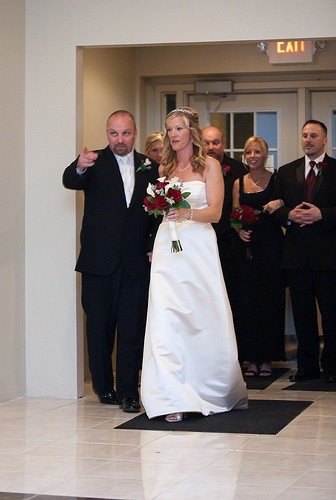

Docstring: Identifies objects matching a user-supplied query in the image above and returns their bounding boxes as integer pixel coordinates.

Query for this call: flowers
[143,176,191,253]
[231,206,262,259]
[316,162,328,174]
[222,165,231,176]
[136,157,152,172]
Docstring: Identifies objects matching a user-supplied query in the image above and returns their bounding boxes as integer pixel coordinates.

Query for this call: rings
[174,214,176,218]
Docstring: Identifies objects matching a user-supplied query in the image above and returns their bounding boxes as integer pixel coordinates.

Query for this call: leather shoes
[119,399,140,412]
[97,389,117,404]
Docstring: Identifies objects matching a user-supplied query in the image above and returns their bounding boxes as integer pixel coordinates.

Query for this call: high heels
[165,412,188,422]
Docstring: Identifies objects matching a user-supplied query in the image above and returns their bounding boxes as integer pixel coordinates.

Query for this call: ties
[120,156,131,204]
[306,161,318,202]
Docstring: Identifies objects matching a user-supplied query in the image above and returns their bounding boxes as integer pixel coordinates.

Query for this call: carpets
[114,400,314,434]
[241,367,291,390]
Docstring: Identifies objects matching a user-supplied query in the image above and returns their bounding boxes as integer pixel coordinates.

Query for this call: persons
[232,136,285,377]
[138,130,167,371]
[140,106,249,422]
[61,109,164,414]
[276,119,336,383]
[199,124,250,313]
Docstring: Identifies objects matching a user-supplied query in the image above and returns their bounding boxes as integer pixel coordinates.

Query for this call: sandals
[244,361,273,377]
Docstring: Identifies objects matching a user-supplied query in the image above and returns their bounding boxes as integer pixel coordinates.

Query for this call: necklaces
[174,162,191,172]
[249,173,265,185]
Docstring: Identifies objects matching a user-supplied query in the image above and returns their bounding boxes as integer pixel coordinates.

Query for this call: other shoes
[289,370,320,382]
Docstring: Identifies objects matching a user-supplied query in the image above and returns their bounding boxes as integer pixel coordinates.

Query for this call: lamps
[257,40,327,65]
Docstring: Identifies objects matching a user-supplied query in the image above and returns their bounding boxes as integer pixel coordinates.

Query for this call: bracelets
[187,208,194,220]
